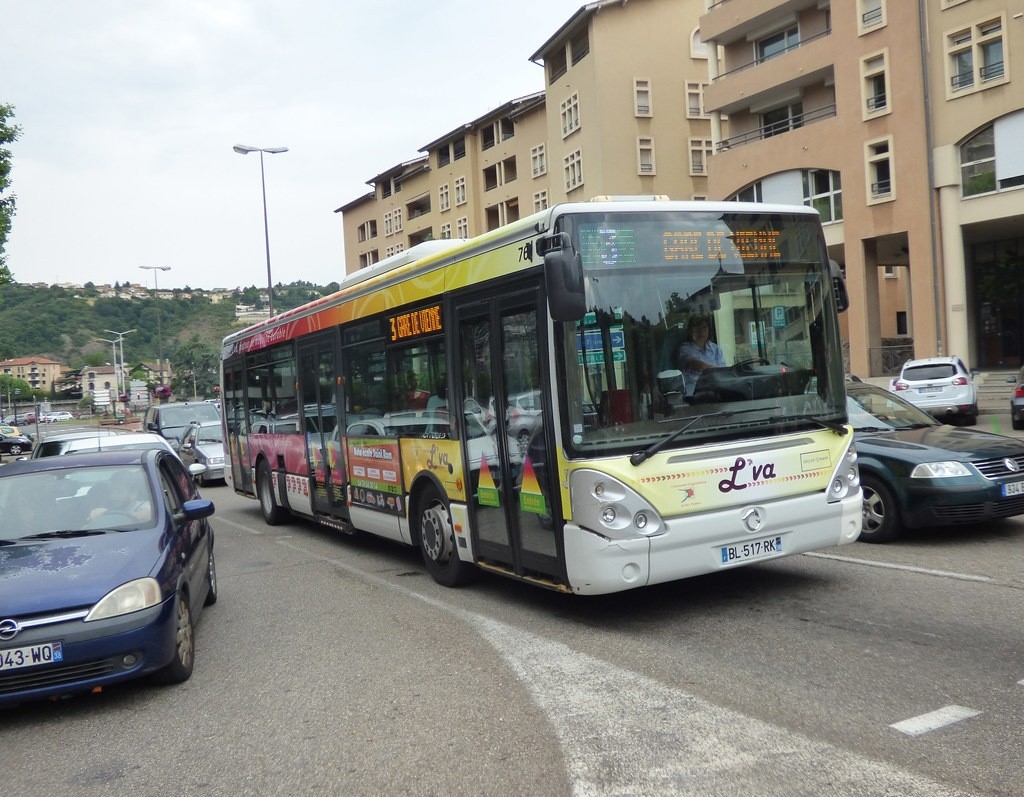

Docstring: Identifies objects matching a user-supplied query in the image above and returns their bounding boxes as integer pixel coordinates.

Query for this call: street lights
[233,144,289,401]
[104,329,136,394]
[139,265,171,386]
[95,338,124,402]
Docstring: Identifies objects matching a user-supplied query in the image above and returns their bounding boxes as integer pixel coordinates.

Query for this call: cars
[1,411,74,425]
[228,389,545,496]
[202,400,222,419]
[803,373,1024,544]
[0,423,33,455]
[0,450,217,706]
[16,429,206,482]
[1006,366,1024,430]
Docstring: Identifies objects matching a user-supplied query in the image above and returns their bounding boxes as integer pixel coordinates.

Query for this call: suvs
[178,421,224,486]
[886,354,980,426]
[143,402,222,455]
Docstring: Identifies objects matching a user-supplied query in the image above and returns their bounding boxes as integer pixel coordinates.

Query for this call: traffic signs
[577,331,625,352]
[577,350,627,364]
[574,309,623,329]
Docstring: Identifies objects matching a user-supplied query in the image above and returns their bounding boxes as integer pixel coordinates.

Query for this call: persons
[89,472,152,523]
[426,373,450,435]
[406,371,427,409]
[675,313,727,405]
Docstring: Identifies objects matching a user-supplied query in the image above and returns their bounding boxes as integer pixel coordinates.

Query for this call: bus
[219,195,862,598]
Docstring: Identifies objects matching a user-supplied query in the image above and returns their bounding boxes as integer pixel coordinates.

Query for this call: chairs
[347,394,485,442]
